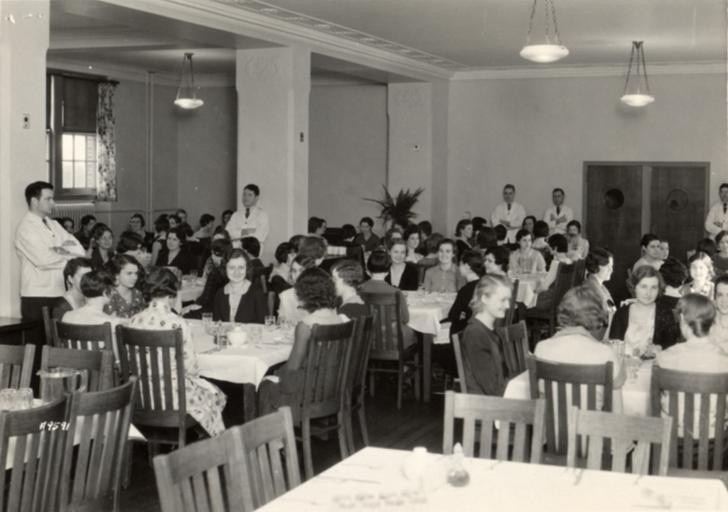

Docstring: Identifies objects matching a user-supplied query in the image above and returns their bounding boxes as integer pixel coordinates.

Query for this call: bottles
[402,446,438,478]
[448,443,470,488]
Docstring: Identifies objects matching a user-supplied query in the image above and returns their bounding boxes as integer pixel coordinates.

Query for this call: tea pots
[36,366,88,430]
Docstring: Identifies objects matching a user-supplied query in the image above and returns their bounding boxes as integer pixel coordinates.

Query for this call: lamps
[174,51,205,110]
[518,0,568,65]
[618,41,655,110]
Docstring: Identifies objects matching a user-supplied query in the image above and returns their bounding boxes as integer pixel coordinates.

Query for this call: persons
[47,184,363,471]
[15,184,87,398]
[345,184,727,472]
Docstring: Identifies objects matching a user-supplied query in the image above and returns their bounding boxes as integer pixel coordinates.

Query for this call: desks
[494,354,727,462]
[254,446,727,511]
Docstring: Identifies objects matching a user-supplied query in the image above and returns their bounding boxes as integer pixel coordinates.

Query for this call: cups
[0,386,35,411]
[609,339,640,381]
[201,310,294,349]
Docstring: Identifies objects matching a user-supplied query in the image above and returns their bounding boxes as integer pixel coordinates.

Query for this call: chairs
[153,426,254,512]
[252,320,349,483]
[308,318,372,447]
[529,354,613,469]
[565,404,676,478]
[116,324,201,494]
[652,364,726,482]
[59,374,137,512]
[0,222,728,392]
[442,390,548,464]
[3,394,64,512]
[236,405,301,510]
[358,290,430,407]
